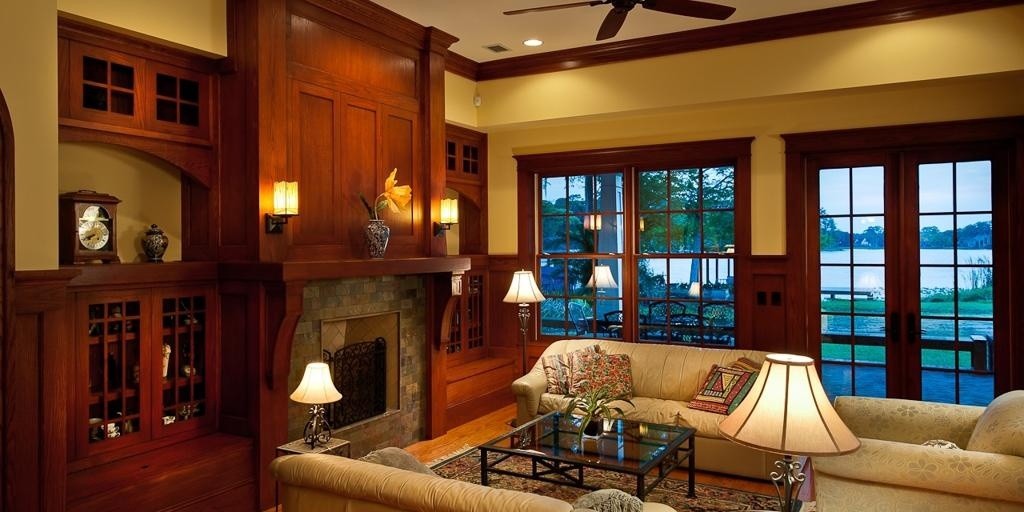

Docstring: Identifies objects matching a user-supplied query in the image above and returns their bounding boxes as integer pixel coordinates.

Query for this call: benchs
[824,329,986,372]
[821,288,873,300]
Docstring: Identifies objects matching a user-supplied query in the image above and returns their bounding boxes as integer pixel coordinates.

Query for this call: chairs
[603,311,647,340]
[697,302,734,345]
[648,299,686,341]
[567,302,606,337]
[664,313,708,343]
[811,387,1023,511]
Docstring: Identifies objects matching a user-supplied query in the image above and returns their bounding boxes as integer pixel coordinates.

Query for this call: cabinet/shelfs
[446,121,488,185]
[445,267,493,370]
[58,8,220,149]
[71,281,218,462]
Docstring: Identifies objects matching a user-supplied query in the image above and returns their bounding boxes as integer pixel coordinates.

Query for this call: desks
[591,317,735,346]
[272,435,352,511]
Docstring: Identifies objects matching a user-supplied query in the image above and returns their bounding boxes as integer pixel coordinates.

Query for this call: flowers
[369,167,413,220]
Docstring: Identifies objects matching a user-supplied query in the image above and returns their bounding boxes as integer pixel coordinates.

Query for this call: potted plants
[562,381,635,447]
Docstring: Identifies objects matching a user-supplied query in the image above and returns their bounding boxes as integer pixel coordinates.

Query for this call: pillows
[566,351,632,400]
[569,487,644,512]
[729,357,762,370]
[686,364,759,416]
[923,438,962,451]
[354,446,442,478]
[540,344,600,395]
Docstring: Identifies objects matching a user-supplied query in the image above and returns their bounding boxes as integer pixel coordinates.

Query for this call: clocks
[58,189,122,264]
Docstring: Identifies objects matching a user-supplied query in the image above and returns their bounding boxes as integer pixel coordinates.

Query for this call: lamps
[639,217,644,232]
[715,353,862,511]
[586,264,618,296]
[588,214,602,231]
[687,281,703,297]
[433,198,458,237]
[263,180,300,232]
[502,268,546,377]
[289,361,345,448]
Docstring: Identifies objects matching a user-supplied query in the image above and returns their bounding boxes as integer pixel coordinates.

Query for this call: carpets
[424,442,817,509]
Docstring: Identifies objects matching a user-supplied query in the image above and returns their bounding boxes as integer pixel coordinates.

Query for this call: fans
[502,0,739,42]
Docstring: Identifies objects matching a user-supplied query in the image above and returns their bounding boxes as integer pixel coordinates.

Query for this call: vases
[362,219,391,260]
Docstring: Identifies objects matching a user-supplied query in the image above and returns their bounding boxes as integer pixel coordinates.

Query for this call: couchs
[268,447,679,512]
[510,337,800,486]
